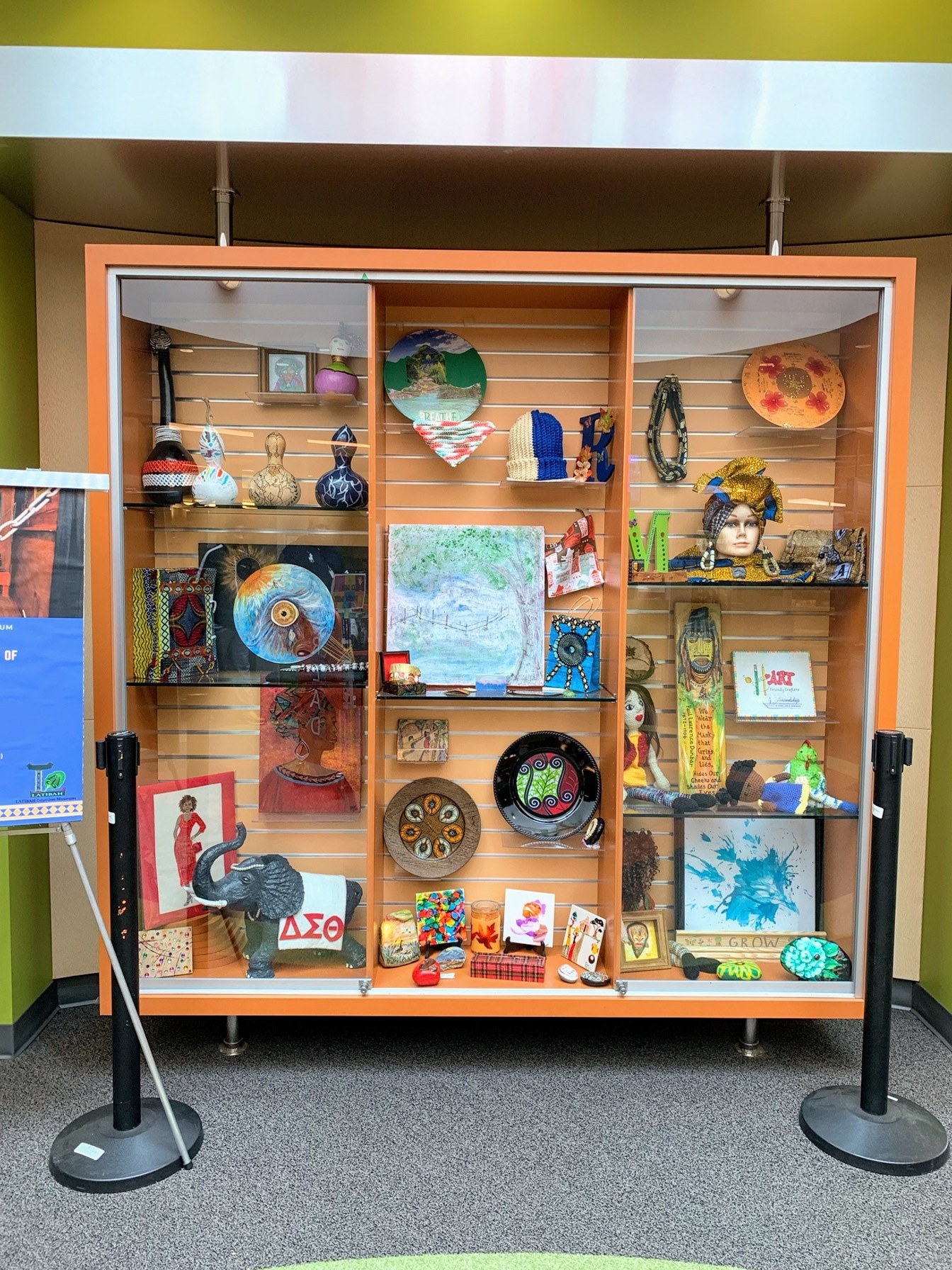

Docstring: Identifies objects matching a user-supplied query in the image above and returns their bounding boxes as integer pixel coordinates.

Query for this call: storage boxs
[471,952,546,983]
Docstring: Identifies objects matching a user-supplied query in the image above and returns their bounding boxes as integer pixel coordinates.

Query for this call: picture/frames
[621,909,672,976]
[258,342,318,393]
[674,806,826,933]
[137,771,240,933]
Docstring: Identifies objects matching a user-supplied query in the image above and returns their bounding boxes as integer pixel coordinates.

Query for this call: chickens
[781,739,827,794]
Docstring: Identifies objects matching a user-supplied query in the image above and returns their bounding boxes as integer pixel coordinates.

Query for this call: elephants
[180,822,367,978]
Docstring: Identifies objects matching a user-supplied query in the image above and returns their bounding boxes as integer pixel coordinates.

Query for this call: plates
[741,342,846,429]
[493,731,602,842]
[384,777,482,878]
[233,564,334,664]
[383,328,486,425]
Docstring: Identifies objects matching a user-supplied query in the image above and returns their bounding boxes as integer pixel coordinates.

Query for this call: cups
[471,900,501,954]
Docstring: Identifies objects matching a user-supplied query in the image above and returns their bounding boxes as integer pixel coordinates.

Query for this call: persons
[716,759,858,815]
[692,456,783,559]
[623,827,722,980]
[623,683,716,811]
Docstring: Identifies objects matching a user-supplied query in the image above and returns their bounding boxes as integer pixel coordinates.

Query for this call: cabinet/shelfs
[84,240,921,1061]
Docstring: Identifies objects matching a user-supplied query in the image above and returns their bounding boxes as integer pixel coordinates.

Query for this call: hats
[506,409,567,480]
